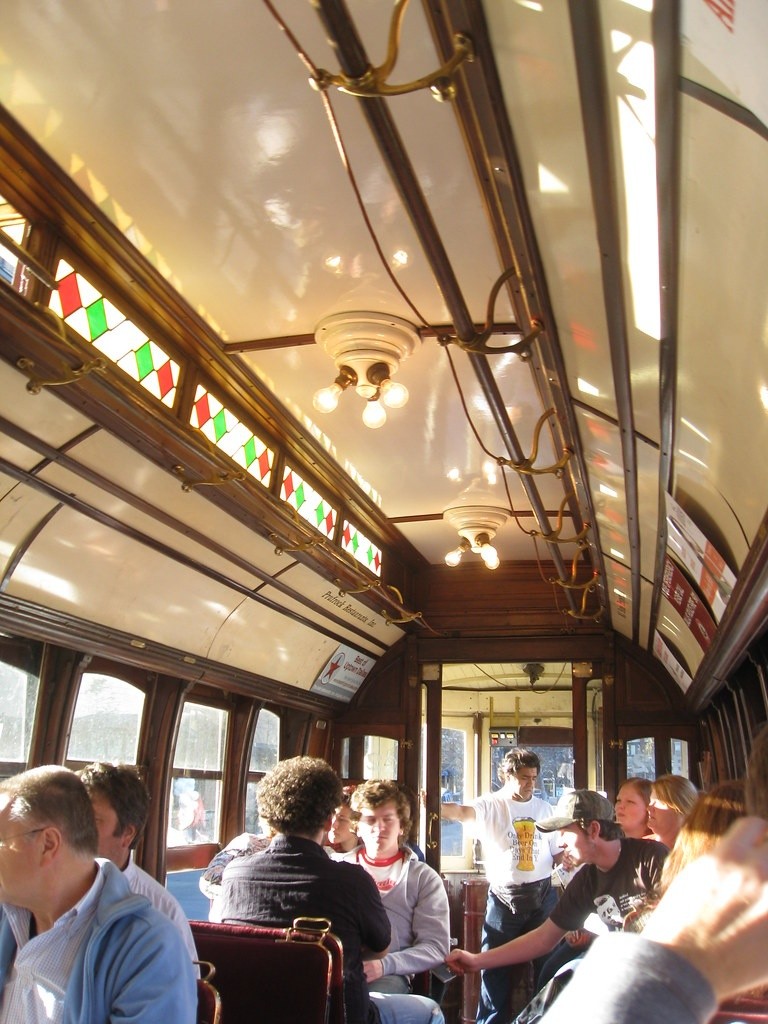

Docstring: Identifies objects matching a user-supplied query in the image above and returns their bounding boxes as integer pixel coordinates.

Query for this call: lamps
[311,311,422,429]
[443,505,511,570]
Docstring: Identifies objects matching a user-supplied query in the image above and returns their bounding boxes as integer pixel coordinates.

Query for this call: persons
[438,748,566,1023]
[209,757,446,1024]
[444,789,671,976]
[331,779,452,996]
[199,779,425,902]
[74,762,202,980]
[0,766,198,1023]
[536,718,768,1024]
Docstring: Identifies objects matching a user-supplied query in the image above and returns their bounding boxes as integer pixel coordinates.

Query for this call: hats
[535,789,614,833]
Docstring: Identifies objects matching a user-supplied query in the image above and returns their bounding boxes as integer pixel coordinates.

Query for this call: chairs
[188,880,451,1024]
